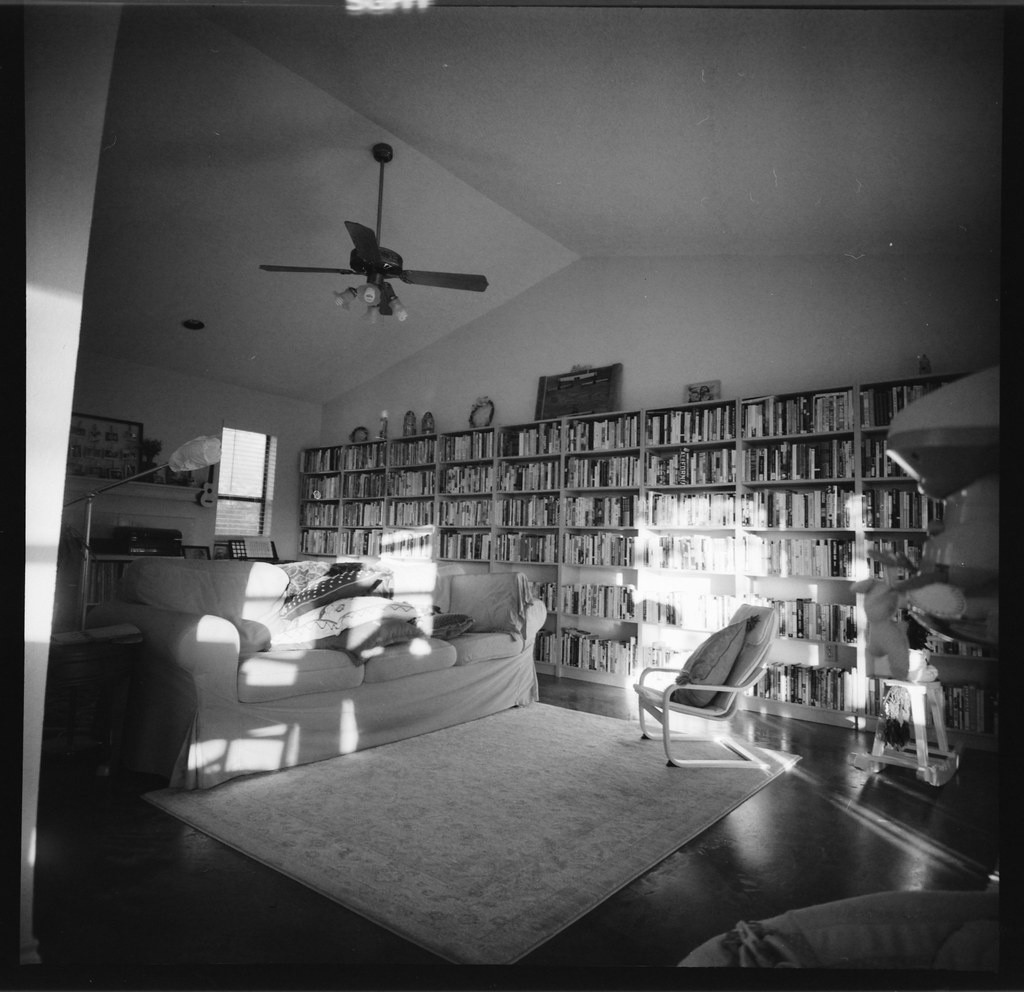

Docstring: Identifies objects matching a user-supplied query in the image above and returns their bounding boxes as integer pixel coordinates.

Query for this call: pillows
[272,596,423,644]
[670,614,762,708]
[419,612,476,639]
[278,566,392,620]
[296,618,427,667]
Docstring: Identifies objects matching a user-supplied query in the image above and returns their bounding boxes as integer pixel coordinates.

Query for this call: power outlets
[824,644,837,662]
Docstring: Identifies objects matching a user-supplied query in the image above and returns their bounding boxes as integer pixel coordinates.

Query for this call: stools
[47,641,140,781]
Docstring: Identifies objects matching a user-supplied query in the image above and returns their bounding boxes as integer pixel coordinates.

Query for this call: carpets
[140,700,804,965]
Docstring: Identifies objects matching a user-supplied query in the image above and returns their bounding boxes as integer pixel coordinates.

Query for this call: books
[302,378,985,733]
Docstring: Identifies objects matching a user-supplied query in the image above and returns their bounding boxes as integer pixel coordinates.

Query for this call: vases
[144,451,154,470]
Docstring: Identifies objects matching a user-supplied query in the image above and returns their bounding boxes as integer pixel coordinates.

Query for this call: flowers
[130,436,163,456]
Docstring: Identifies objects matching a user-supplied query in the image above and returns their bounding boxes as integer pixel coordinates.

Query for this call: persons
[698,385,714,402]
[687,391,700,403]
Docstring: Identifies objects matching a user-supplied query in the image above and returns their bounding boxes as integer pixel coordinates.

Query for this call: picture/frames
[104,447,120,461]
[124,430,138,442]
[182,546,211,560]
[85,446,103,459]
[108,468,124,481]
[682,379,722,403]
[89,430,102,442]
[105,432,119,442]
[87,464,105,477]
[67,462,85,475]
[122,448,137,460]
[534,362,623,420]
[72,425,88,439]
[124,464,137,480]
[70,443,84,459]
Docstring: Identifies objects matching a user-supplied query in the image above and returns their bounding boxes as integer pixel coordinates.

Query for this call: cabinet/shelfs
[298,370,1000,757]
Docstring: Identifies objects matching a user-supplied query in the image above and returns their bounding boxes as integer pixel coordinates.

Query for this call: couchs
[82,557,548,792]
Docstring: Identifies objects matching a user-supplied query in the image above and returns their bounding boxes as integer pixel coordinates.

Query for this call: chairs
[631,603,780,770]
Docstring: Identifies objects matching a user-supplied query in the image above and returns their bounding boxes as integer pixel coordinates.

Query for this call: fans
[260,143,490,317]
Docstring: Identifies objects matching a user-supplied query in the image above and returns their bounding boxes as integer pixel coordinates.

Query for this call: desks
[75,554,184,636]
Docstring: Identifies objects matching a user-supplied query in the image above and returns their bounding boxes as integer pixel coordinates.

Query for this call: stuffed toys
[850,576,966,680]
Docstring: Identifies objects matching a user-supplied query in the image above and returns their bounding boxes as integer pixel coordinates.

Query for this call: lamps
[332,245,408,323]
[63,434,222,639]
